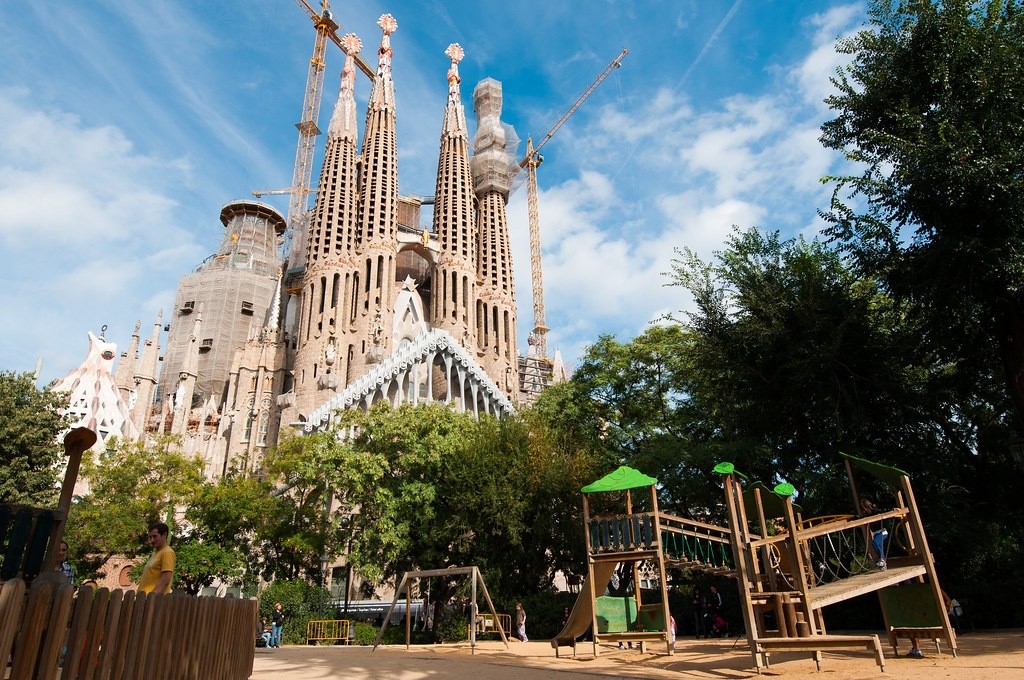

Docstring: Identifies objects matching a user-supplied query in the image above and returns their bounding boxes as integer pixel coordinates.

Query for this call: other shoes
[906,649,923,656]
[482,631,487,635]
[618,645,626,651]
[628,646,633,649]
[276,645,279,648]
[265,645,271,648]
[876,559,887,571]
[271,646,274,648]
[520,637,529,642]
[696,634,699,639]
[704,634,708,638]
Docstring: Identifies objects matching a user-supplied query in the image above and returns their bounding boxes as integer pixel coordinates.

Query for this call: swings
[412,576,431,633]
[449,574,481,625]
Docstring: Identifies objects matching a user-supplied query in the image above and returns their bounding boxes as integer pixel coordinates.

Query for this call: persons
[690,585,709,639]
[467,597,487,636]
[269,602,285,648]
[847,498,902,571]
[663,611,677,653]
[560,606,570,632]
[335,611,434,633]
[899,579,924,657]
[619,641,635,650]
[54,541,73,592]
[941,589,961,636]
[135,523,176,599]
[706,584,723,639]
[515,601,529,643]
[256,616,273,649]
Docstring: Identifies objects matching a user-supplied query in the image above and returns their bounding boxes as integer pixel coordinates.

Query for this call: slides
[551,550,617,647]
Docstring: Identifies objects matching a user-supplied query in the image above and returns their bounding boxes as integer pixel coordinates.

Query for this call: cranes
[513,46,631,356]
[253,0,376,272]
